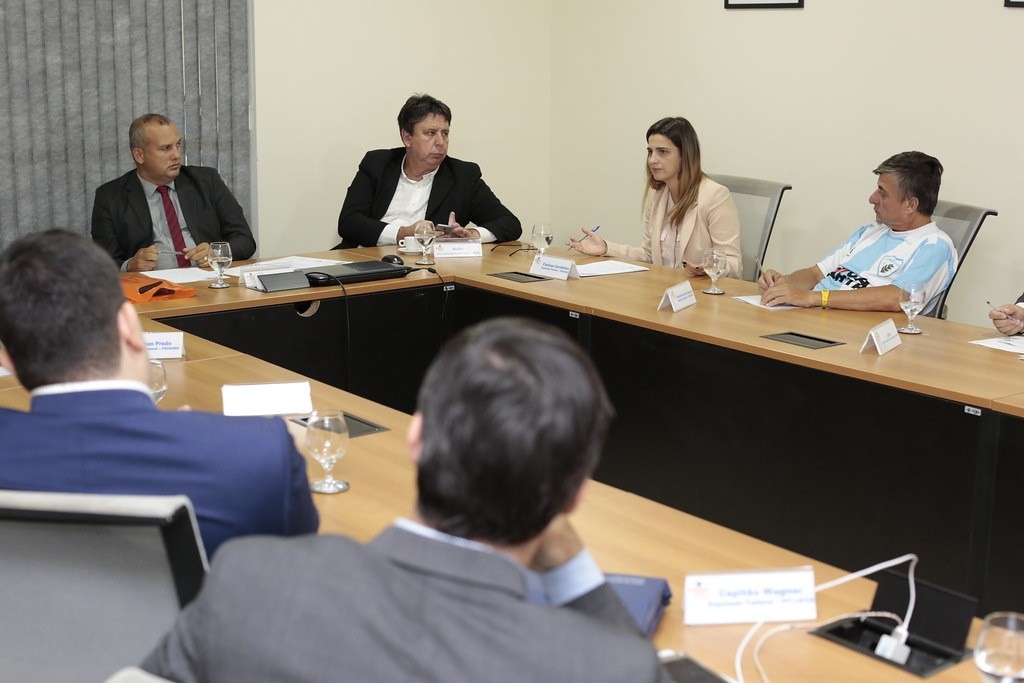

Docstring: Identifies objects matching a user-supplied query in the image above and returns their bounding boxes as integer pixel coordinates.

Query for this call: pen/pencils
[569,226,600,251]
[158,249,187,255]
[755,256,766,277]
[987,300,1012,318]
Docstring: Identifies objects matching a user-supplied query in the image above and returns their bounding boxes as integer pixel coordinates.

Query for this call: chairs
[0,488,209,683]
[705,173,793,285]
[922,199,997,319]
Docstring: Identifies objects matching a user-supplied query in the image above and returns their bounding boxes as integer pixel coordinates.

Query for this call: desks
[0,238,1024,683]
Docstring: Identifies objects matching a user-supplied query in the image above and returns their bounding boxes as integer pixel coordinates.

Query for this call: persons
[330,94,523,250]
[140,320,675,683]
[91,113,256,272]
[0,227,319,560]
[567,116,744,278]
[989,293,1024,337]
[757,151,958,314]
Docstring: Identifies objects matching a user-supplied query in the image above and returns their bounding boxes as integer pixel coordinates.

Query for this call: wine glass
[207,241,233,289]
[702,248,727,295]
[896,281,925,334]
[531,223,554,254]
[302,408,350,494]
[414,220,436,265]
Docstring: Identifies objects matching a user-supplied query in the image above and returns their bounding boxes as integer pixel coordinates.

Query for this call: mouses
[381,255,404,265]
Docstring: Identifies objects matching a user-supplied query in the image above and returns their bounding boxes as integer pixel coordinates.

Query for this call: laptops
[304,259,412,286]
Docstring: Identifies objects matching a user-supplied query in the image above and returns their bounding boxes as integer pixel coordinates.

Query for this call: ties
[157,185,194,268]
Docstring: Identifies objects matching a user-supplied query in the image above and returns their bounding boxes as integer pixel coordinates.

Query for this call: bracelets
[821,289,829,309]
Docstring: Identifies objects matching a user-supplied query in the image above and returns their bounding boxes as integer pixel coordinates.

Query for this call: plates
[398,248,423,255]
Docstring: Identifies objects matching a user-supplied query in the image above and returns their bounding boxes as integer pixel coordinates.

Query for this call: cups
[399,237,420,251]
[148,359,169,404]
[973,611,1024,682]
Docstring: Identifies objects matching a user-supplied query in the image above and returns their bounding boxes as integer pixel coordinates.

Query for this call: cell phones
[436,224,452,234]
